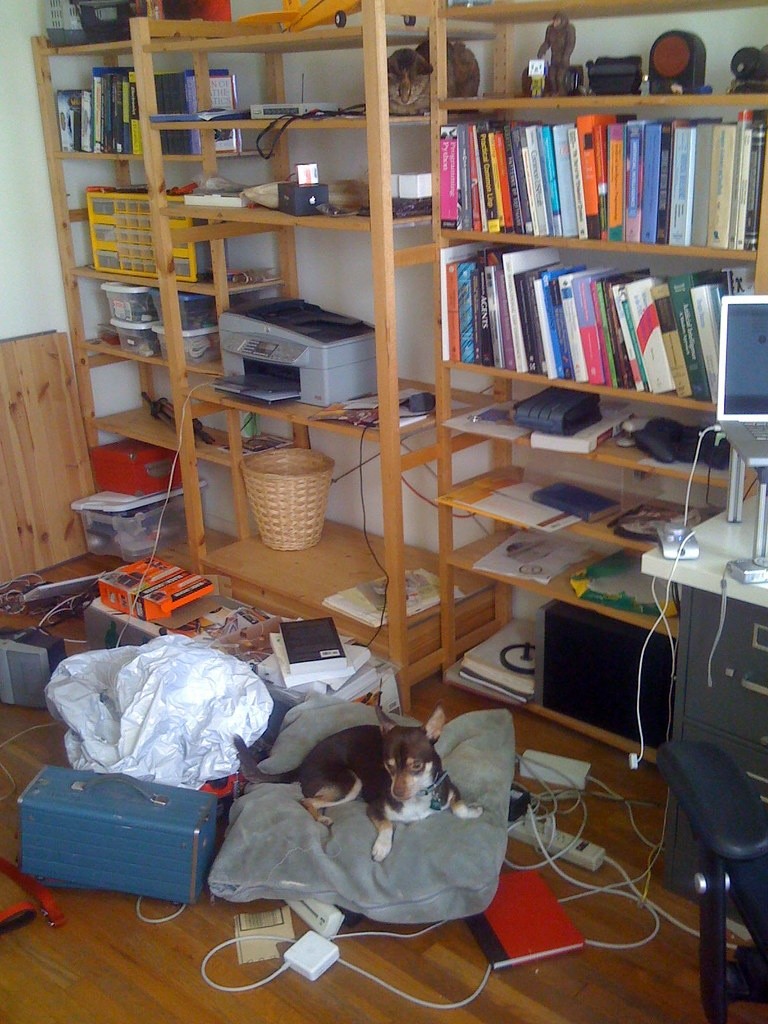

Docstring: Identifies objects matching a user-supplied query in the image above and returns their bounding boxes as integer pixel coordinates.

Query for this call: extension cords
[284,897,344,938]
[506,814,606,872]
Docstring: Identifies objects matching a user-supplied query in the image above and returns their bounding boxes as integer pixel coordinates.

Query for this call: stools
[651,738,768,1024]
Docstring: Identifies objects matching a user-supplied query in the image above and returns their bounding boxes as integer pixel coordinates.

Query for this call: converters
[520,750,592,790]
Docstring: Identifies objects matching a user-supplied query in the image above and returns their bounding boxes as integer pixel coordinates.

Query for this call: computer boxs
[536,599,678,752]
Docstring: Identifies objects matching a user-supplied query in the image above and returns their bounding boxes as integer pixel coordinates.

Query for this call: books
[466,871,583,969]
[460,619,536,703]
[439,110,766,405]
[218,436,295,456]
[531,408,635,454]
[279,617,347,675]
[321,567,465,628]
[470,483,620,533]
[136,0,231,39]
[56,67,243,155]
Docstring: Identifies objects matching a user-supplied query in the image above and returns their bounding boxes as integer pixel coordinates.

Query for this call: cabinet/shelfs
[29,0,767,893]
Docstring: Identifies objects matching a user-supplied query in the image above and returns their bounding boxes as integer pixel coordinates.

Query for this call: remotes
[656,522,699,559]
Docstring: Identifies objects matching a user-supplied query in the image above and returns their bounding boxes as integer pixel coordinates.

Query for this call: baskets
[236,447,336,552]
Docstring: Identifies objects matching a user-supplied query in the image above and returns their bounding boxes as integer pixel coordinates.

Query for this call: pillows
[207,687,517,924]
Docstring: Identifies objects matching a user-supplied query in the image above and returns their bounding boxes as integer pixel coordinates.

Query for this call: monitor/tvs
[717,295,768,421]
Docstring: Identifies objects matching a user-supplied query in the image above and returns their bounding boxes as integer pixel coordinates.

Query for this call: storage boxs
[98,555,232,630]
[18,759,220,908]
[88,437,181,497]
[69,479,209,564]
[81,592,279,667]
[100,277,259,362]
[533,600,680,752]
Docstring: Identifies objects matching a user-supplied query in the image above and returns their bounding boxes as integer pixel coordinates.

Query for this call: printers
[214,299,378,409]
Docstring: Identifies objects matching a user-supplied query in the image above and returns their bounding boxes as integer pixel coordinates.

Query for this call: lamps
[651,420,768,562]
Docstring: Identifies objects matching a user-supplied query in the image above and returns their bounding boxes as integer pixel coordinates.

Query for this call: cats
[389,39,480,116]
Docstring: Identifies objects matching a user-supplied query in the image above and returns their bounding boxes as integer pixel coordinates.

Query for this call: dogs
[233,698,485,862]
